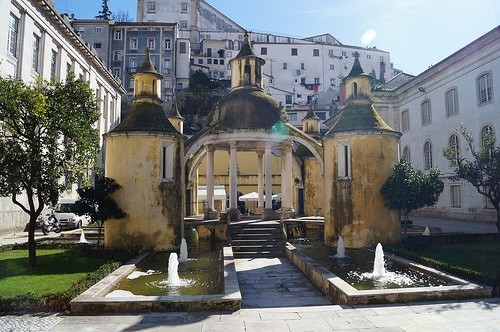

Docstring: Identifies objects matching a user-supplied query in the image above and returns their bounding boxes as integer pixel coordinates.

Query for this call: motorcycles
[40,213,63,235]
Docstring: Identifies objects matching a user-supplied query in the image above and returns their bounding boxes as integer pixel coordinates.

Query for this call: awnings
[238,191,266,214]
[272,193,281,199]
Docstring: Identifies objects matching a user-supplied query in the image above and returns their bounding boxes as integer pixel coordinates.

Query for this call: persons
[246,207,252,216]
[272,199,281,211]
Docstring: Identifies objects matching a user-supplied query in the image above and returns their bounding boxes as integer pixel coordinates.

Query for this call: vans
[54,199,92,229]
